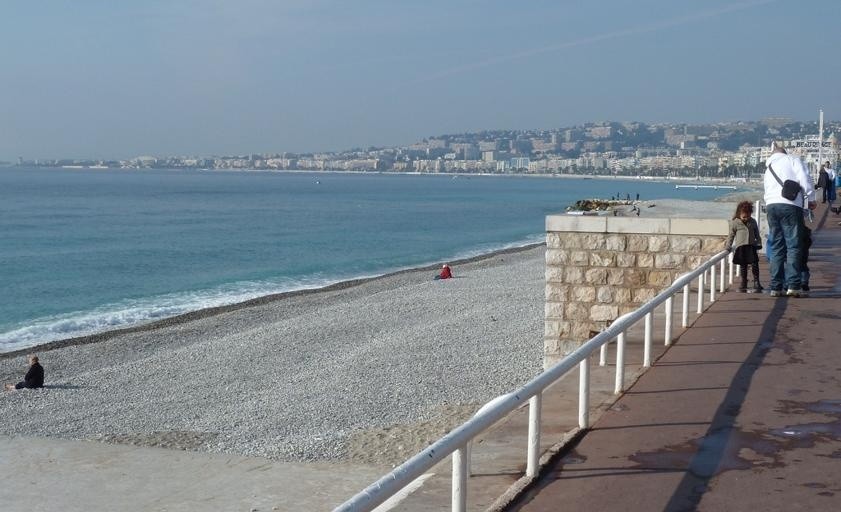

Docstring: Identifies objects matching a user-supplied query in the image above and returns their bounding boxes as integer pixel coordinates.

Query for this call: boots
[735,278,764,293]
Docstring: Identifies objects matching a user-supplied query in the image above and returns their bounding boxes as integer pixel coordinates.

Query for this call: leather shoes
[820,199,831,203]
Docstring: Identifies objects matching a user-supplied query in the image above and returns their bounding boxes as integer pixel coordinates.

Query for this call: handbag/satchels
[781,179,800,201]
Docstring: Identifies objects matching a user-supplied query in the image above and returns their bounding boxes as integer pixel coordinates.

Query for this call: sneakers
[769,286,811,298]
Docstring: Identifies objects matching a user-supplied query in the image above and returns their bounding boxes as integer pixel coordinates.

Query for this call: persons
[434,264,453,280]
[726,147,816,297]
[5,356,44,391]
[817,161,836,203]
